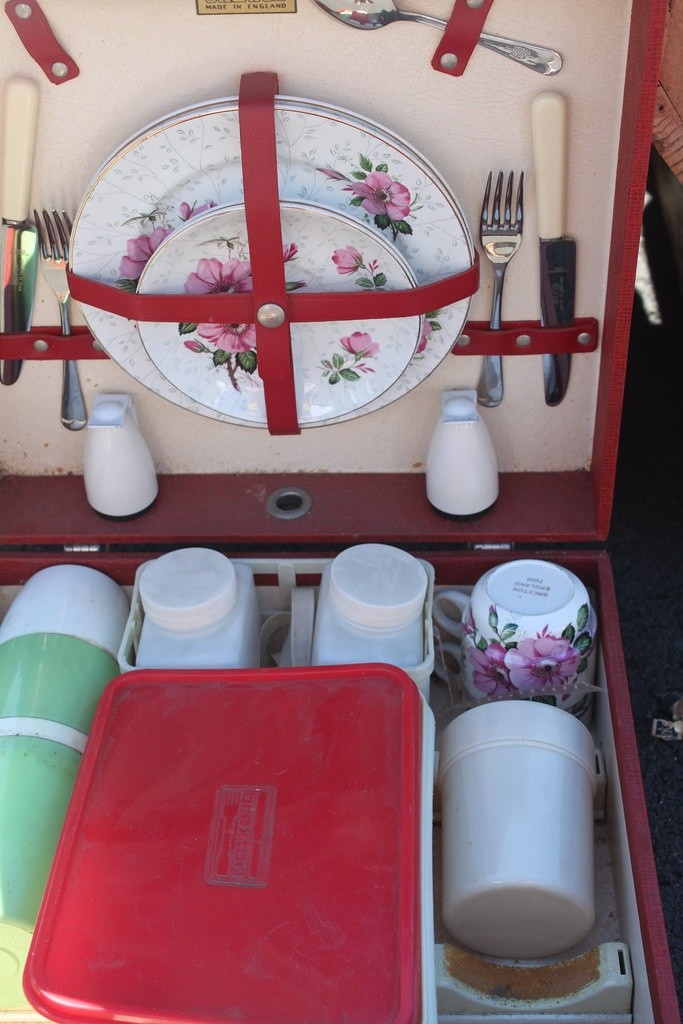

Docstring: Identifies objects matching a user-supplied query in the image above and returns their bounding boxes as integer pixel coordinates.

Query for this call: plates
[67,93,476,431]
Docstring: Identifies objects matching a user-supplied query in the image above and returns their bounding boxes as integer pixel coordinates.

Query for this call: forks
[469,169,525,411]
[31,207,89,431]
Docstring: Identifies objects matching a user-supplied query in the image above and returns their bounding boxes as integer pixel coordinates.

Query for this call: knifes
[533,89,577,406]
[0,73,36,385]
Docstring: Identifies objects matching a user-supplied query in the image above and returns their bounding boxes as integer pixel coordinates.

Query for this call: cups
[432,700,594,955]
[0,565,129,1011]
[434,559,597,727]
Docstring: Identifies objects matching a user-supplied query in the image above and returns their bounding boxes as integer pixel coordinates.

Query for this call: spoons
[314,0,562,76]
